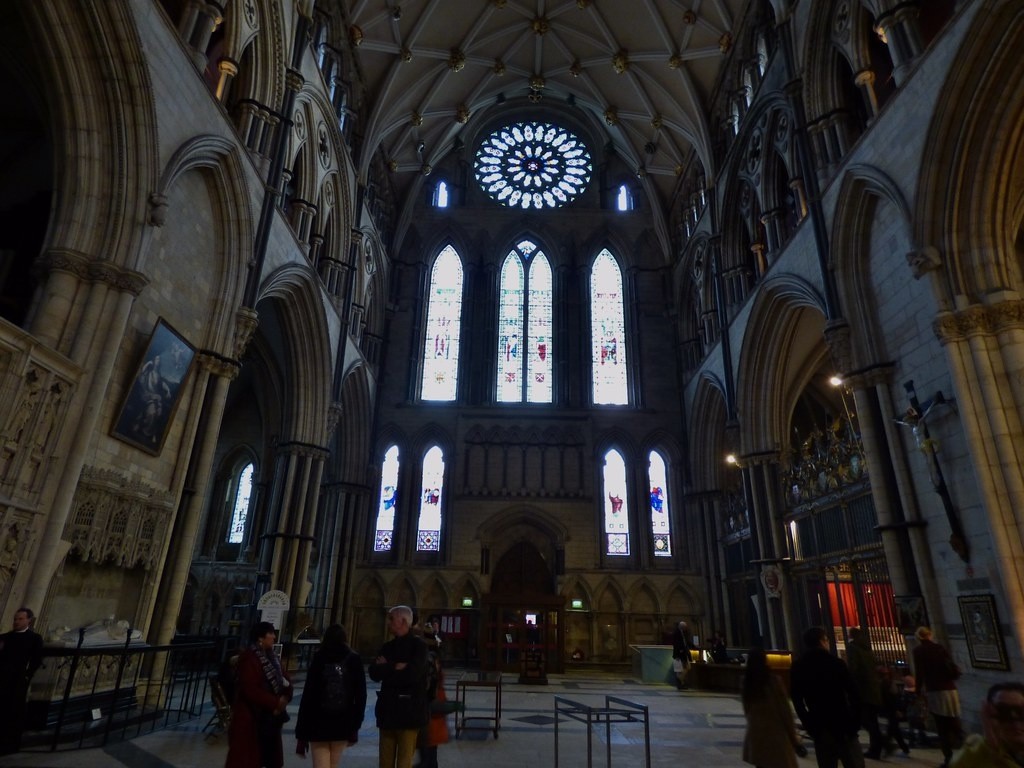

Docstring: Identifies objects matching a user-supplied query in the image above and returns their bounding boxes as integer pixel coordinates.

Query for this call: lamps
[725,449,781,464]
[829,360,896,387]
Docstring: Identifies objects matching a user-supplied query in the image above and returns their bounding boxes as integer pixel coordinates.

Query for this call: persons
[844,629,909,759]
[11,381,37,443]
[903,676,928,745]
[790,628,866,768]
[672,621,692,689]
[712,630,728,656]
[422,622,439,651]
[36,389,61,449]
[0,607,43,689]
[912,424,941,494]
[224,622,293,768]
[295,624,367,768]
[1,539,18,591]
[369,605,427,768]
[433,618,447,648]
[915,626,965,768]
[743,651,807,768]
[951,681,1024,768]
[413,652,450,768]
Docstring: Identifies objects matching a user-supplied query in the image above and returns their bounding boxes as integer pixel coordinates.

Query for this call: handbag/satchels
[944,661,962,681]
[673,658,684,672]
[324,650,357,714]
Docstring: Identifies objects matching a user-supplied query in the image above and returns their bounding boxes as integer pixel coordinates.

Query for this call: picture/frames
[957,595,1010,672]
[109,315,200,458]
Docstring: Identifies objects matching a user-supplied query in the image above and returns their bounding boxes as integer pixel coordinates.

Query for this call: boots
[413,745,438,768]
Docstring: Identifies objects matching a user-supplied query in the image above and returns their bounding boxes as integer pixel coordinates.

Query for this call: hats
[422,622,436,645]
[914,627,933,640]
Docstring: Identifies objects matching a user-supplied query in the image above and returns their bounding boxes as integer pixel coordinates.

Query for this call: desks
[454,669,503,739]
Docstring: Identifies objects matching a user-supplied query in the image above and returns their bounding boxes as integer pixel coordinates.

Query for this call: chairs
[203,675,232,740]
